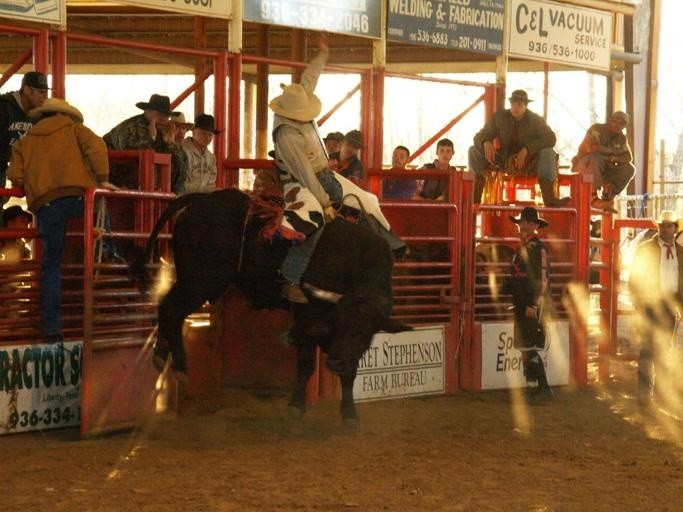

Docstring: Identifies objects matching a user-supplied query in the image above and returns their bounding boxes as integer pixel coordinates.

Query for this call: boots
[538,177,570,207]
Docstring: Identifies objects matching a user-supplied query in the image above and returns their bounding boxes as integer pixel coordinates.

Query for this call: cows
[126,188,412,430]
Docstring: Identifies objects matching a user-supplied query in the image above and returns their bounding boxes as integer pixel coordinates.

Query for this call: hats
[135,94,225,136]
[612,111,629,127]
[655,210,683,234]
[512,90,533,102]
[270,84,322,122]
[3,205,32,226]
[509,207,548,228]
[29,97,84,123]
[22,72,55,92]
[323,130,363,149]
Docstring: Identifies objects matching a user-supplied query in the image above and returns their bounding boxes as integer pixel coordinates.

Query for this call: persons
[573,112,636,213]
[0,71,54,169]
[270,32,369,304]
[6,97,121,343]
[383,146,416,198]
[414,139,457,200]
[468,90,571,206]
[625,210,682,405]
[167,110,222,196]
[102,93,181,263]
[253,150,283,197]
[501,208,554,406]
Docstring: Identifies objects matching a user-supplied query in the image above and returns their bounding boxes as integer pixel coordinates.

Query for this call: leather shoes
[592,196,613,209]
[279,283,309,305]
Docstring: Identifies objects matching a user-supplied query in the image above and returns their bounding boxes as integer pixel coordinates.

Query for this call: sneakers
[528,389,554,404]
